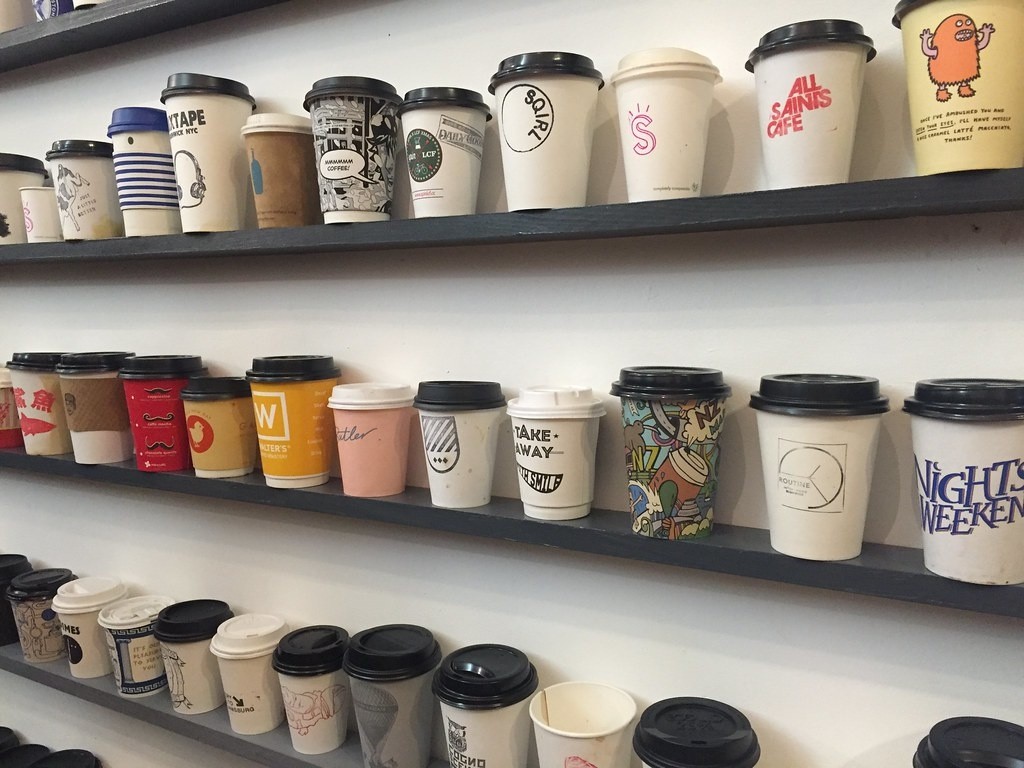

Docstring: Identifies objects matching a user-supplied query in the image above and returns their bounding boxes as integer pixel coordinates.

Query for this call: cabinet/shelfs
[0,0,1024,768]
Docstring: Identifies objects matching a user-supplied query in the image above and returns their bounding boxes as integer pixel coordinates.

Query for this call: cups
[0,0,1024,244]
[0,548,1024,768]
[0,351,1024,595]
[0,726,104,768]
